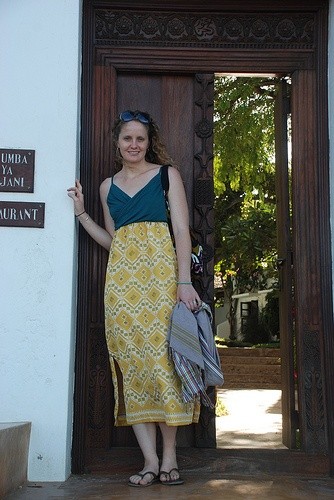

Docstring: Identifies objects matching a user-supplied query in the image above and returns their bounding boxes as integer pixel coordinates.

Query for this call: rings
[192,300,197,304]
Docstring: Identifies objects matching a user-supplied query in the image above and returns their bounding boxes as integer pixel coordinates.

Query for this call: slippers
[128,471,161,486]
[160,468,184,485]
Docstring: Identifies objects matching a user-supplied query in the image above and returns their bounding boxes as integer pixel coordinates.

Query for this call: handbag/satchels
[188,227,205,276]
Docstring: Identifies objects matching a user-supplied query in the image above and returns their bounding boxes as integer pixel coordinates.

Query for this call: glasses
[117,110,150,124]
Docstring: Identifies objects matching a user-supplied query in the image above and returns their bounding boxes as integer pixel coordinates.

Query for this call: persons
[67,110,202,487]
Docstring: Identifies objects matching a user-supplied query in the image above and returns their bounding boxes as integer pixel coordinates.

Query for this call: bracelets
[75,210,86,217]
[175,281,192,285]
[79,215,89,224]
